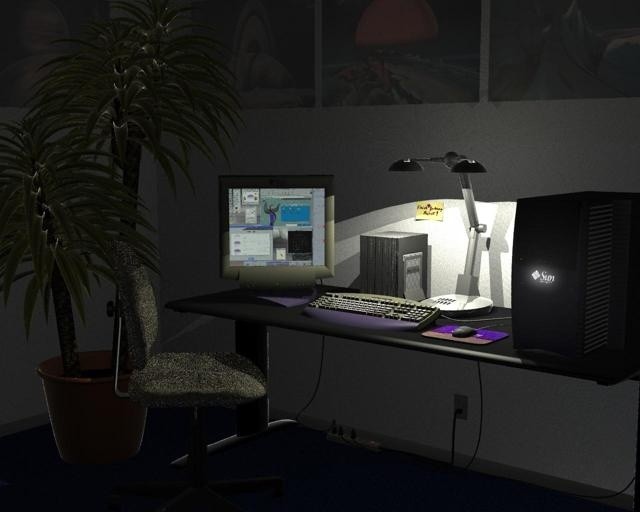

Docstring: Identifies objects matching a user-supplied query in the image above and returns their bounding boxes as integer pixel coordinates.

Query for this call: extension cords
[325,429,373,449]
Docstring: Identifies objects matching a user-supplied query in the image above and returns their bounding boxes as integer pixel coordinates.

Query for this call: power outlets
[452,394,468,421]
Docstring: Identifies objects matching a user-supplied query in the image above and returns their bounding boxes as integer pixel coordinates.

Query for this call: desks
[163,282,640,512]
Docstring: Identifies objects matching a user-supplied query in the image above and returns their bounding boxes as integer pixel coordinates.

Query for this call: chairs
[95,238,288,511]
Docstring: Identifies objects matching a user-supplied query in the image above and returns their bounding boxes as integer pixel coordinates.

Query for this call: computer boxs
[513,192,639,377]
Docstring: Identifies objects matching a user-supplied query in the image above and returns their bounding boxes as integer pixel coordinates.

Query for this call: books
[357,232,428,298]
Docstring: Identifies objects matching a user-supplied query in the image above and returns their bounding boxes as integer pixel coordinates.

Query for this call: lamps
[386,149,490,297]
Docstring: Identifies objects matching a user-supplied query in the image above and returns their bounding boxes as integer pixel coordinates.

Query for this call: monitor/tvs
[219,175,334,296]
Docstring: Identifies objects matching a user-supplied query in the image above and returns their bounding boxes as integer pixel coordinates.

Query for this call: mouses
[452,325,476,337]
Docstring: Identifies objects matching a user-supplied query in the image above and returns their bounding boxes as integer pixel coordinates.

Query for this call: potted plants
[0,0,258,470]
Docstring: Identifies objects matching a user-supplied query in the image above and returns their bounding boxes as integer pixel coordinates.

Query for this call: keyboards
[309,291,440,330]
